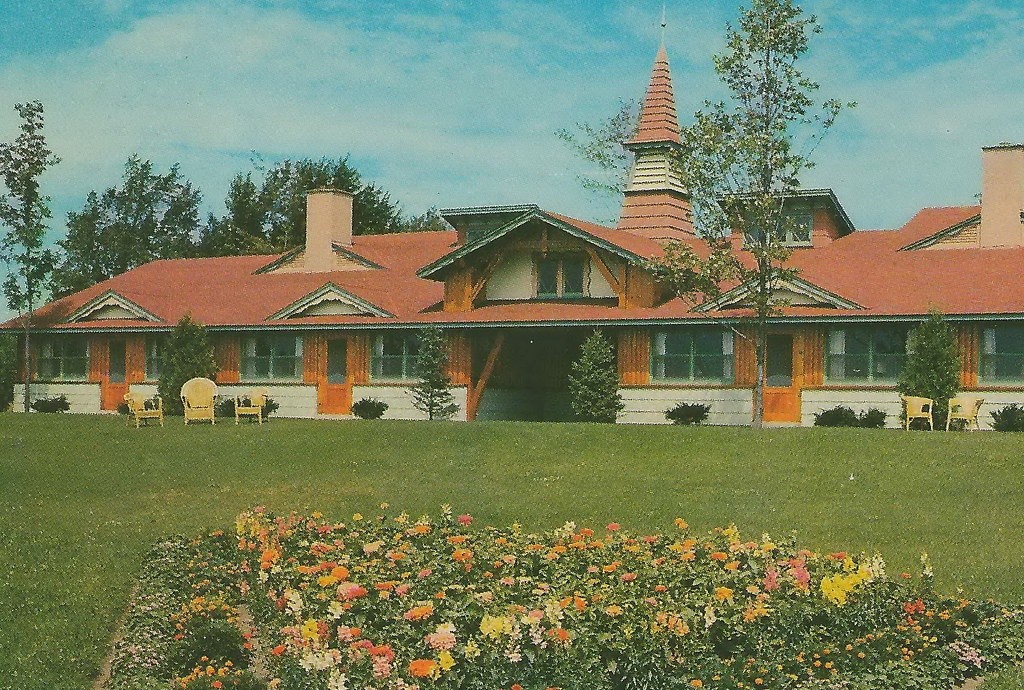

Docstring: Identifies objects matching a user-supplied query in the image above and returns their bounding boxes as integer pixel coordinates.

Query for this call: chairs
[124,392,163,428]
[180,378,218,425]
[235,388,269,426]
[901,396,933,431]
[946,396,984,432]
